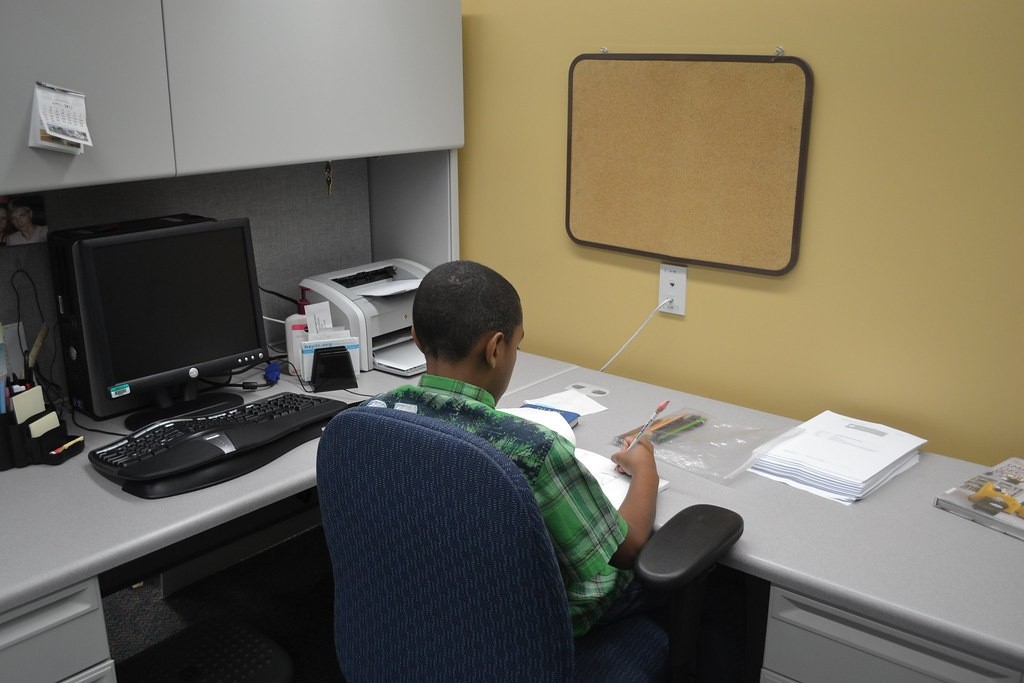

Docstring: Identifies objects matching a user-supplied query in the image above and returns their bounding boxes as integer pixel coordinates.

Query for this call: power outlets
[659,265,687,316]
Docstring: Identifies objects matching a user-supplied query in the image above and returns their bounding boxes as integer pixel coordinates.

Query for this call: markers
[48,434,84,456]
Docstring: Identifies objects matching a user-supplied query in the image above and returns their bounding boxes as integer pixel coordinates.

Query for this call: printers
[303,258,432,378]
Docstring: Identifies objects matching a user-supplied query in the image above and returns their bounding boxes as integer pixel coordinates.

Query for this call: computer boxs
[45,212,218,421]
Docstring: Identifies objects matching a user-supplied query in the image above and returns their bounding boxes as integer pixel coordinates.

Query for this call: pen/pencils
[612,399,670,473]
[4,349,38,412]
[615,406,708,448]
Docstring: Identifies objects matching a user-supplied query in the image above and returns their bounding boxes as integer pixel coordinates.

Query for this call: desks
[0,336,581,615]
[479,366,1024,672]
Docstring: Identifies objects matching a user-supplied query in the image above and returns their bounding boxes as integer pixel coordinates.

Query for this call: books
[11,385,60,438]
[494,404,670,511]
[933,457,1024,541]
[28,81,93,155]
[301,337,359,382]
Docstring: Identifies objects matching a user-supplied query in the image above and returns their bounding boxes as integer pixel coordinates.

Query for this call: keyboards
[89,391,348,481]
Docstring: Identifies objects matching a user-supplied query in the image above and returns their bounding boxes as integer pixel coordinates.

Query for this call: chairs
[315,406,745,682]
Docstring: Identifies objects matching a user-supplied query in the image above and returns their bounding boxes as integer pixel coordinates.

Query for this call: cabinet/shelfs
[0,0,177,195]
[0,564,117,683]
[161,0,462,178]
[760,584,1024,683]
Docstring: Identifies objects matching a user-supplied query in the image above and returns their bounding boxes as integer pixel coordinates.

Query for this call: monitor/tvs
[72,217,269,432]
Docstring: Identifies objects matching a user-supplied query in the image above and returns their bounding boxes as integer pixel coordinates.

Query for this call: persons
[0,199,48,245]
[358,259,705,683]
